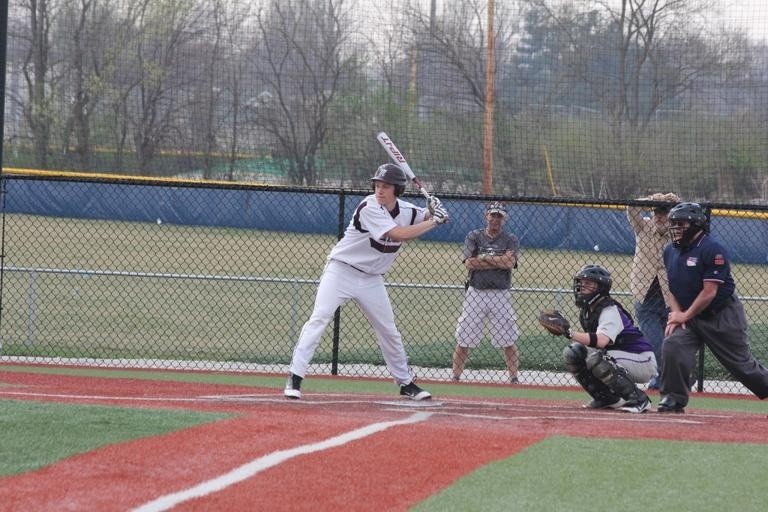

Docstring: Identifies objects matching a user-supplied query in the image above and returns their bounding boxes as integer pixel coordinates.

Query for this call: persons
[449,201,520,384]
[626,192,695,392]
[284,163,449,403]
[538,264,657,414]
[657,201,768,413]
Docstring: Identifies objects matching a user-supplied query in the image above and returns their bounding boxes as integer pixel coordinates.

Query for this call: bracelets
[648,195,654,201]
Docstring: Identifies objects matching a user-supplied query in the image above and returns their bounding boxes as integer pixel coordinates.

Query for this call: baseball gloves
[538,310,570,336]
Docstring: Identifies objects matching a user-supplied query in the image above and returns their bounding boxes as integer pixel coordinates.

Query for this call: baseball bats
[377,131,450,224]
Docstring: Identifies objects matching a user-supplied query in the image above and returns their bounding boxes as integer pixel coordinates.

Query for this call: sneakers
[657,396,685,413]
[400,381,432,401]
[582,397,652,413]
[283,372,304,399]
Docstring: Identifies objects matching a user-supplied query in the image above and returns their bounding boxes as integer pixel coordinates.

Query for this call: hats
[486,201,507,216]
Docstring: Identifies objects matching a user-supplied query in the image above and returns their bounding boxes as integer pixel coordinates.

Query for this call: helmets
[574,263,613,308]
[667,202,710,248]
[369,163,408,186]
